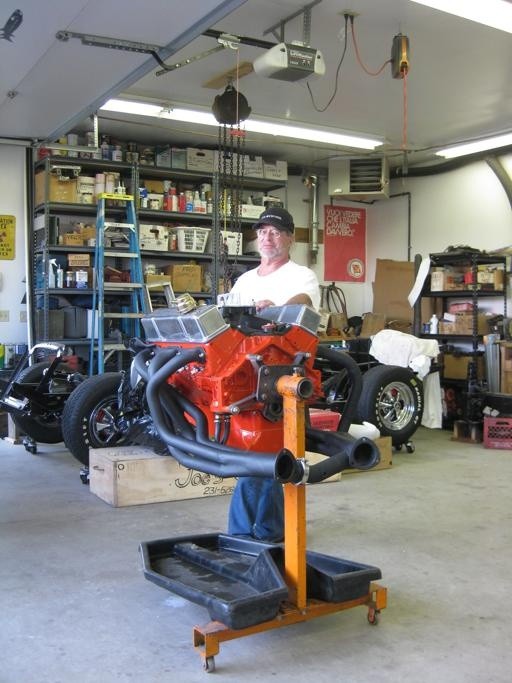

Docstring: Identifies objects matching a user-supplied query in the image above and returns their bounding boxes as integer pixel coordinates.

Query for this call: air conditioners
[328,156,390,200]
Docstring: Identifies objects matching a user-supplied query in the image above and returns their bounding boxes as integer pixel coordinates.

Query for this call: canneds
[169,234,177,252]
[141,198,159,211]
[66,270,76,288]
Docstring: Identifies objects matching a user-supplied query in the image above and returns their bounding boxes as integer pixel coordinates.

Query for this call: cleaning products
[49,259,58,289]
[139,188,147,207]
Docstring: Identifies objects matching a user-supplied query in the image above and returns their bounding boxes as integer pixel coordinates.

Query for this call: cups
[59,134,68,158]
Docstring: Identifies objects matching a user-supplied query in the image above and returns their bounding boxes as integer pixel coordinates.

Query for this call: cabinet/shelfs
[29,156,288,374]
[414,253,506,431]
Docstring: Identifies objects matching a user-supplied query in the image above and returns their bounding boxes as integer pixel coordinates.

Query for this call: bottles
[177,190,214,213]
[429,313,438,334]
[227,196,233,217]
[55,264,76,290]
[101,140,110,161]
[95,173,126,208]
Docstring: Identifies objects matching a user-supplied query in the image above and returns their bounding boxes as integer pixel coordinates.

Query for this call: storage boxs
[444,354,485,380]
[156,147,289,181]
[438,313,492,335]
[477,270,504,291]
[58,227,96,246]
[431,272,464,291]
[164,265,203,292]
[143,274,171,292]
[34,309,65,338]
[142,181,164,193]
[34,170,76,204]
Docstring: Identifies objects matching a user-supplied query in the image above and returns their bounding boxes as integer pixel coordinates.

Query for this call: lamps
[99,97,393,150]
[433,131,512,159]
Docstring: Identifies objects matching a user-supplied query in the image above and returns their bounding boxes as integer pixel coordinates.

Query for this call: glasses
[256,228,288,238]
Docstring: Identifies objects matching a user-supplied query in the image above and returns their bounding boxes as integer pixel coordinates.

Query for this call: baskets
[169,225,212,254]
[482,411,511,451]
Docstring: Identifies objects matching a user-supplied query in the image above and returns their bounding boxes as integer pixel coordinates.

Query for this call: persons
[218,205,322,546]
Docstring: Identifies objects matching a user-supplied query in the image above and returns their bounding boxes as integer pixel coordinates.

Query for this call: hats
[252,207,294,233]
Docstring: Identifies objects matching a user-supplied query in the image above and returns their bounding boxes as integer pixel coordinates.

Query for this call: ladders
[90,193,147,378]
